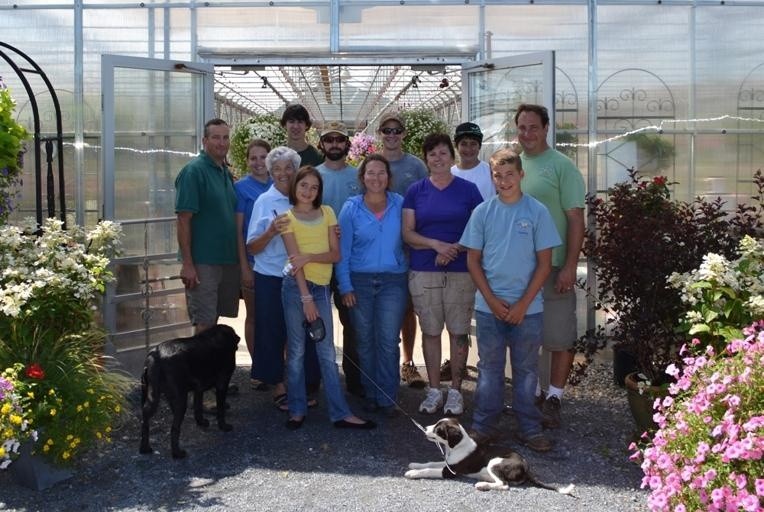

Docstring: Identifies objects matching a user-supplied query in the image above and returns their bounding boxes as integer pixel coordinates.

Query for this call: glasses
[381,127,403,134]
[323,136,346,142]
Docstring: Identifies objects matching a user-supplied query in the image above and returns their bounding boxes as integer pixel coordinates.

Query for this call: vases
[6,415,91,495]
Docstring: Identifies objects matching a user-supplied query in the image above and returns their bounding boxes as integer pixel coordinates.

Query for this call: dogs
[403,417,575,496]
[137,323,240,460]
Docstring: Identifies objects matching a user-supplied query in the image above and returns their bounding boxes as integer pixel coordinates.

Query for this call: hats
[380,112,403,126]
[320,121,348,137]
[454,122,483,139]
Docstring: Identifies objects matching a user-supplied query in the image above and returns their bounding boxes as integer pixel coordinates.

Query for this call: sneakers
[467,428,500,445]
[542,395,561,428]
[402,360,424,387]
[203,399,229,413]
[440,360,467,381]
[502,390,545,416]
[444,388,464,416]
[214,383,238,395]
[419,388,444,414]
[514,432,551,451]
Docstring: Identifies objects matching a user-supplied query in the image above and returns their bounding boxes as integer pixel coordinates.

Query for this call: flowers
[0,72,130,479]
[561,165,764,511]
[226,106,452,177]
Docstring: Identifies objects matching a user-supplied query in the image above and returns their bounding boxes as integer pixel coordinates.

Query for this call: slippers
[272,392,288,411]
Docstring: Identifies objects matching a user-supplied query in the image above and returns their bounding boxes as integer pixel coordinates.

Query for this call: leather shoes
[287,416,304,431]
[333,419,377,429]
